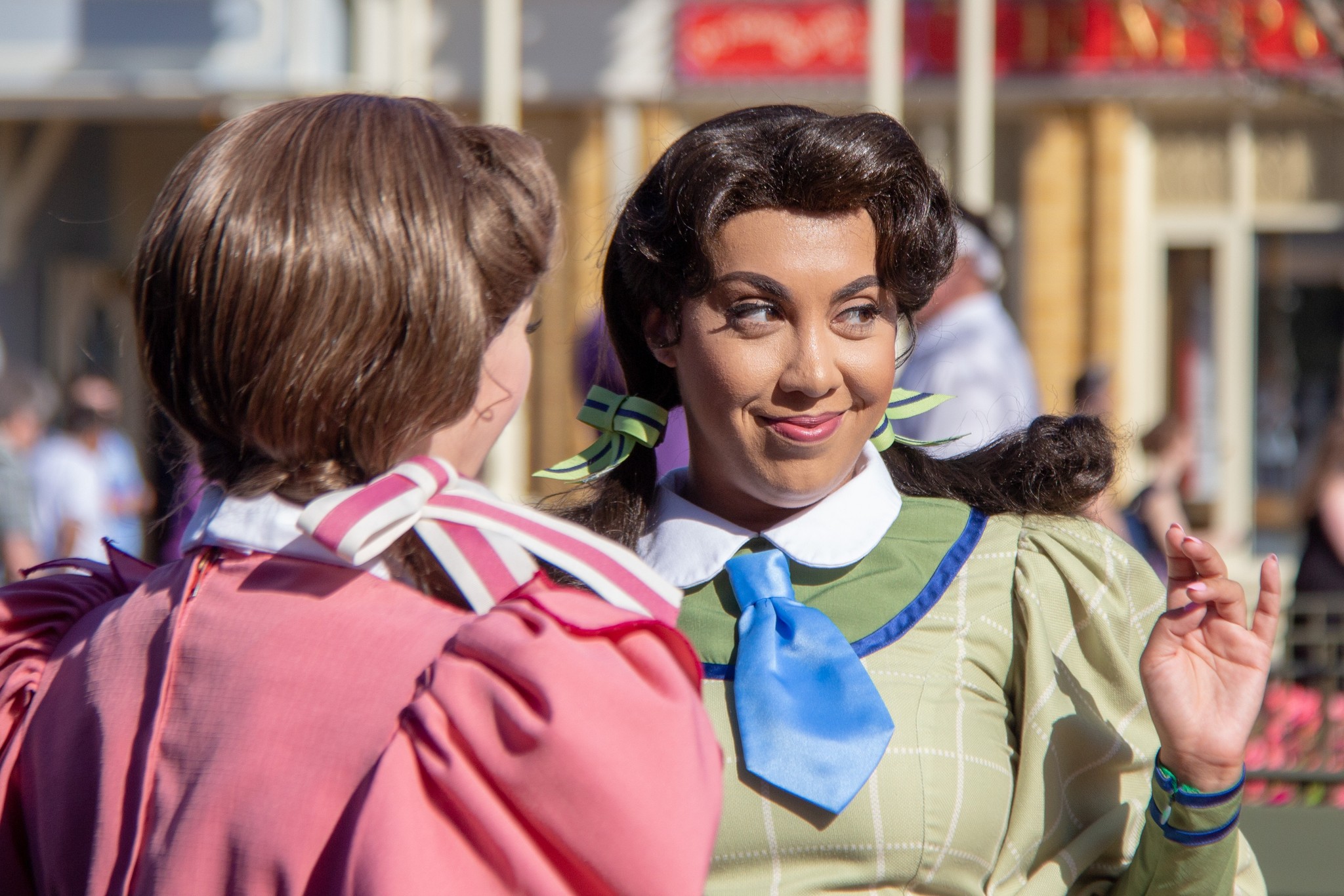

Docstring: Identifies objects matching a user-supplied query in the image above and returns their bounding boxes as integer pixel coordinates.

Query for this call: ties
[724,548,894,815]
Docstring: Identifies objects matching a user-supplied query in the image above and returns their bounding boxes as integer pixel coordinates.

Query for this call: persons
[0,358,65,584]
[18,376,155,575]
[0,94,726,895]
[890,198,1038,455]
[571,314,690,485]
[1285,391,1344,695]
[534,102,1278,896]
[1121,415,1190,588]
[1068,365,1130,546]
